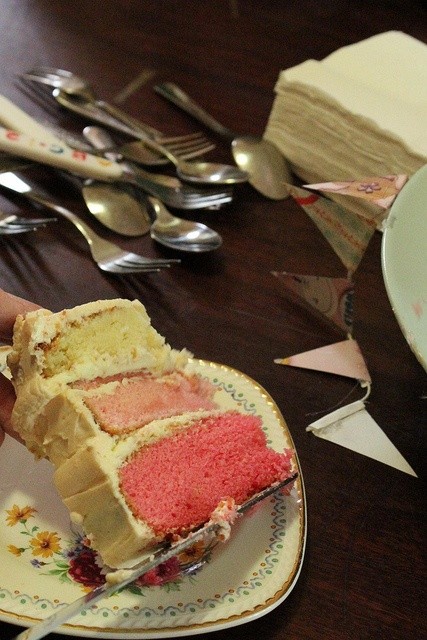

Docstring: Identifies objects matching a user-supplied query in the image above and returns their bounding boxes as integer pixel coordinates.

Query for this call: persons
[0,289,57,457]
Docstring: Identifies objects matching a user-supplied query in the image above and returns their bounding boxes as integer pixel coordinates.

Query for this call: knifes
[16,472,298,640]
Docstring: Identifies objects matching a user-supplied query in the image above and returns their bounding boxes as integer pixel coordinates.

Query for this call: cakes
[9,299,295,574]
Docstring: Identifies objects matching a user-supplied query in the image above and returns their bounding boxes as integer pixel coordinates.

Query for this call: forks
[1,169,181,279]
[71,129,217,167]
[1,212,59,235]
[21,65,152,143]
[0,120,233,211]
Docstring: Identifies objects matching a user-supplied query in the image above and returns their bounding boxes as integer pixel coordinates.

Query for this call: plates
[381,165,427,370]
[0,357,308,639]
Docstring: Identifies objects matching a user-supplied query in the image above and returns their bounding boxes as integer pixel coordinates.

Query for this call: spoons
[0,94,152,239]
[153,79,293,205]
[82,126,229,260]
[54,90,250,186]
